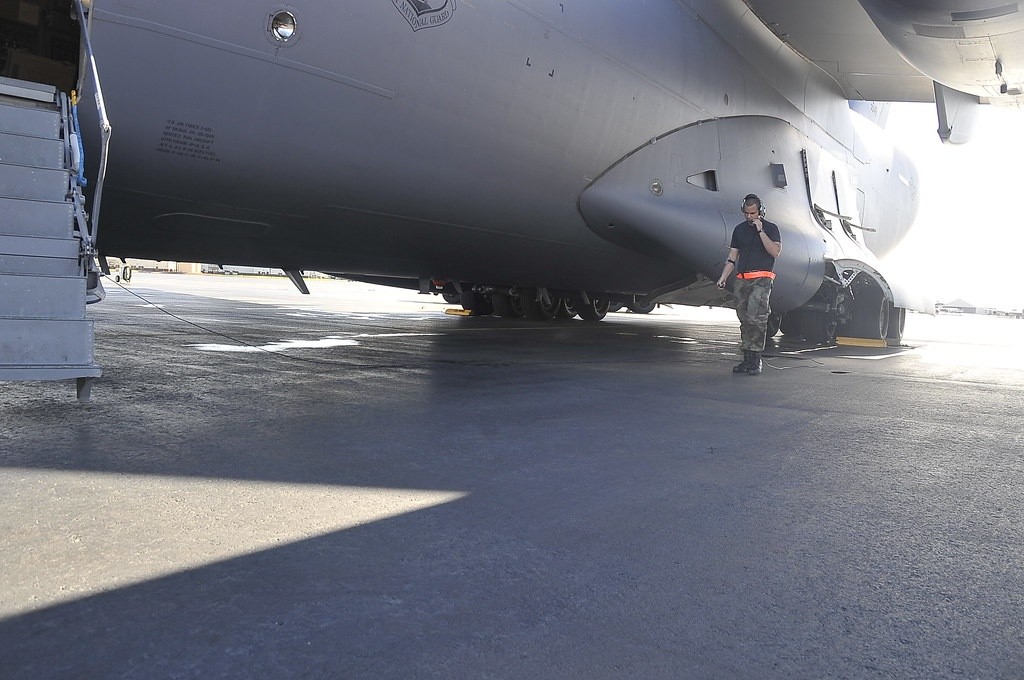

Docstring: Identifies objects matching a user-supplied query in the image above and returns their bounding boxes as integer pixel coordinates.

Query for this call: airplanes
[0,0,1024,320]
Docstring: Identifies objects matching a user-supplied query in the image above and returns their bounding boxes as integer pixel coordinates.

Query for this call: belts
[737,270,776,279]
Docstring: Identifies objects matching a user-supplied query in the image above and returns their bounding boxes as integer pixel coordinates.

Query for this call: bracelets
[758,228,763,234]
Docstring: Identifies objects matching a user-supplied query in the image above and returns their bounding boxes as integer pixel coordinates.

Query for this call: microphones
[751,221,754,223]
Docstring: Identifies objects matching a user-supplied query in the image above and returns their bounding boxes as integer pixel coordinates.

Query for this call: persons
[717,194,781,374]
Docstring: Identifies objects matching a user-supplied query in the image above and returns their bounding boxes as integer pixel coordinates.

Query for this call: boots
[733,351,763,375]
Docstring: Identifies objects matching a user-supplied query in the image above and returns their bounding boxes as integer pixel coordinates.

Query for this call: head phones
[740,194,766,218]
